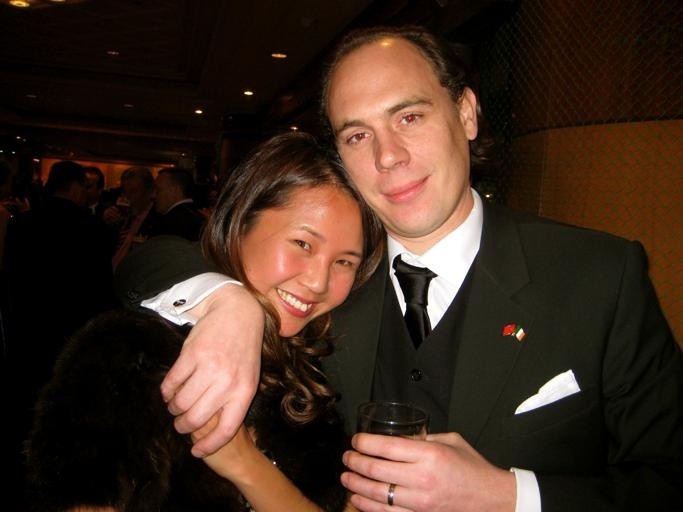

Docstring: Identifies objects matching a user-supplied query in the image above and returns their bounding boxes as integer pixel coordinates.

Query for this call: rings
[387,482,394,506]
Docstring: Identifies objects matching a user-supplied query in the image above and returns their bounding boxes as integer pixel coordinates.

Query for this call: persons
[138,21,682,511]
[1,156,200,357]
[20,128,389,511]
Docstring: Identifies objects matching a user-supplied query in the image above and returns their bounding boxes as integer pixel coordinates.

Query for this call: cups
[357,401,431,444]
[116,198,131,217]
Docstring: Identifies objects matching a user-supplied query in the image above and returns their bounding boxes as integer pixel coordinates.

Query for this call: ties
[392,254,438,350]
